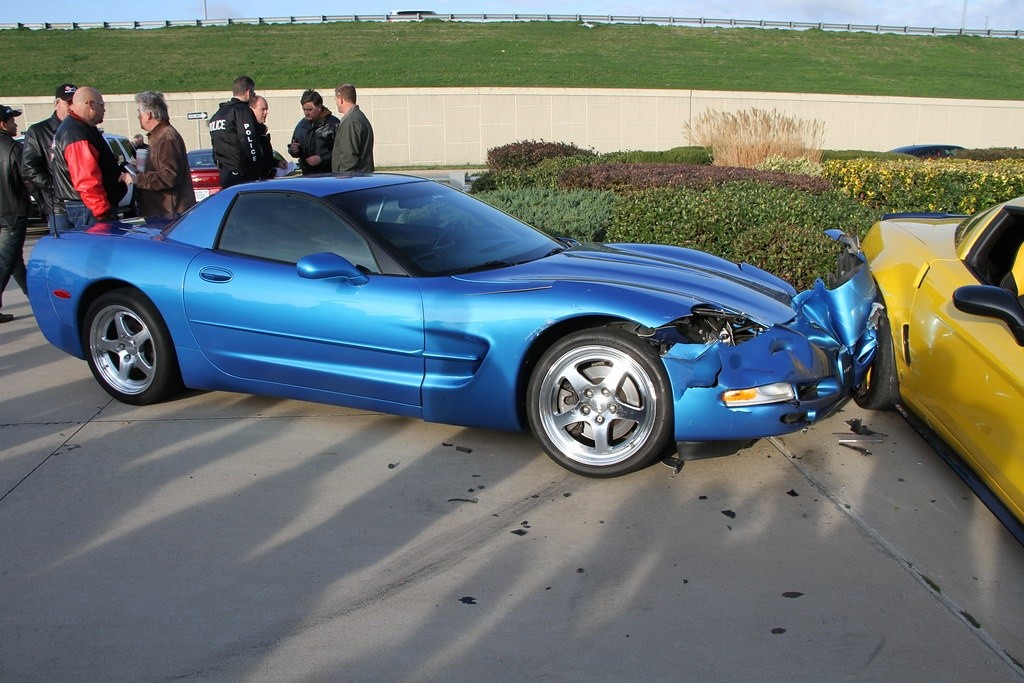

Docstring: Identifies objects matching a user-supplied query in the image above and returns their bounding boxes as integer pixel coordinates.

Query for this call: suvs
[386,10,449,21]
[15,129,137,222]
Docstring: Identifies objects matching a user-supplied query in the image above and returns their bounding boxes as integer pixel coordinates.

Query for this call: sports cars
[853,194,1024,544]
[26,170,880,480]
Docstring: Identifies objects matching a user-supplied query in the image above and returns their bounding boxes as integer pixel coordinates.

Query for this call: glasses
[250,90,256,97]
[85,99,106,108]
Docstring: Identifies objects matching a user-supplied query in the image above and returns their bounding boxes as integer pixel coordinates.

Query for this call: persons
[209,75,263,191]
[51,86,128,230]
[331,83,375,219]
[133,134,148,159]
[117,91,196,225]
[288,89,340,175]
[250,95,287,181]
[0,104,31,323]
[21,83,79,233]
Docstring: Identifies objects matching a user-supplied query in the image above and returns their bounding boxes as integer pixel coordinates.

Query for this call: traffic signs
[186,112,208,120]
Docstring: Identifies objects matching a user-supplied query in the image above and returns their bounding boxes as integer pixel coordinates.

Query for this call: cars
[884,144,965,156]
[188,148,292,202]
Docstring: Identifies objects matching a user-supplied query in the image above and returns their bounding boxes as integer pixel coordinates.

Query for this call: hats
[56,83,78,101]
[0,104,22,121]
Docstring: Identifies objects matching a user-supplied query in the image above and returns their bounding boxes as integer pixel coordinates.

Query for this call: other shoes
[0,313,13,323]
